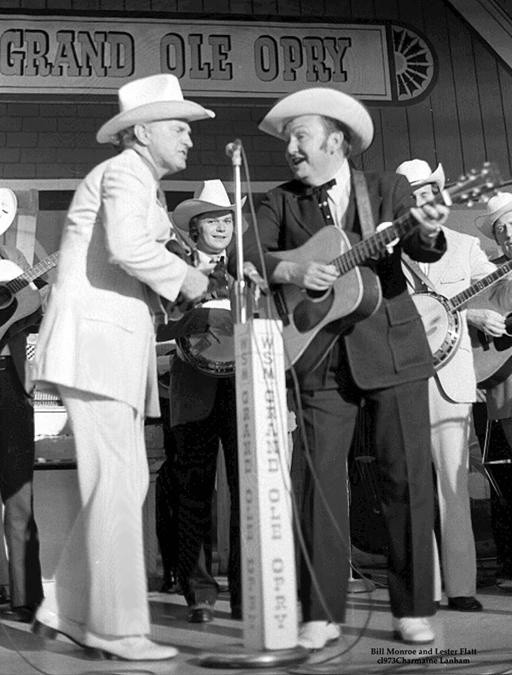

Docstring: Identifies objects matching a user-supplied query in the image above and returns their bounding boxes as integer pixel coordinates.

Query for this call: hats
[258,88,376,157]
[474,192,511,241]
[396,159,446,191]
[172,179,247,231]
[95,73,216,144]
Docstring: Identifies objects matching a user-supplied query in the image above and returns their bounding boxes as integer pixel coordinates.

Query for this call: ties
[312,180,335,225]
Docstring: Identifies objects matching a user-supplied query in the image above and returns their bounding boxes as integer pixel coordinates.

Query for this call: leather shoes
[191,608,214,623]
[449,596,482,612]
[33,606,86,650]
[298,620,340,651]
[390,615,434,644]
[81,628,180,661]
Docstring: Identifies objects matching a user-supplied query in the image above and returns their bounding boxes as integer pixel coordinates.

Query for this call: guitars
[467,274,512,388]
[0,250,59,350]
[265,161,504,380]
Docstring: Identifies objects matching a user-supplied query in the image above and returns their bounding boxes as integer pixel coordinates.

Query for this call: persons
[395,155,500,612]
[0,185,49,621]
[157,397,221,593]
[163,176,250,625]
[26,69,219,664]
[225,85,451,654]
[476,190,512,590]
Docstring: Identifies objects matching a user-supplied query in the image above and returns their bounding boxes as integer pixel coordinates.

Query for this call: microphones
[224,137,245,159]
